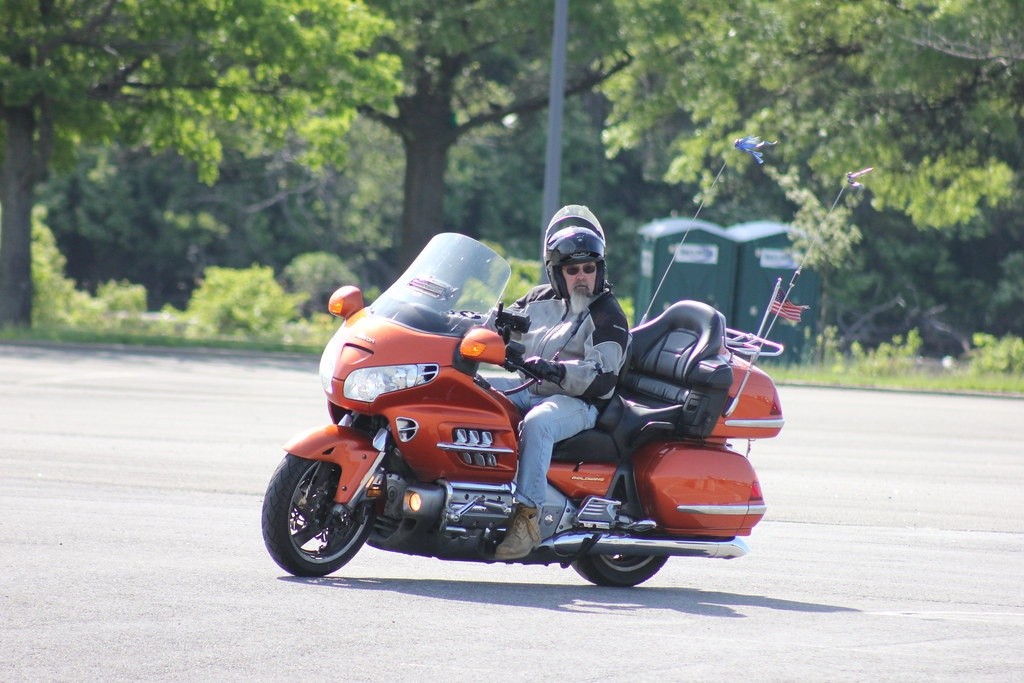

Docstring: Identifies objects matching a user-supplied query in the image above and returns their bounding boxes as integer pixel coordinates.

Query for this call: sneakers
[495,503,542,559]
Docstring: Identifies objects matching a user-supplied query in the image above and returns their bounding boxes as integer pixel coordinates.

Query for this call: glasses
[565,265,596,275]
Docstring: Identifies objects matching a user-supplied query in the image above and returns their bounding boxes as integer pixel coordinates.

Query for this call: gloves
[524,356,566,391]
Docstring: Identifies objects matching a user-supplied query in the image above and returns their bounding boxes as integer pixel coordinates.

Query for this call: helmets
[544,226,606,300]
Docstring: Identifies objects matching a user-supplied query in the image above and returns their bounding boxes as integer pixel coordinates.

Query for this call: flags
[770,287,810,321]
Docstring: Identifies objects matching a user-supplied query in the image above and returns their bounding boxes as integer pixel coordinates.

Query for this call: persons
[440,225,628,560]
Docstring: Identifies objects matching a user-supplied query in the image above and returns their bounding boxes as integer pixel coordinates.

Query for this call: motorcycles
[260,231,855,590]
